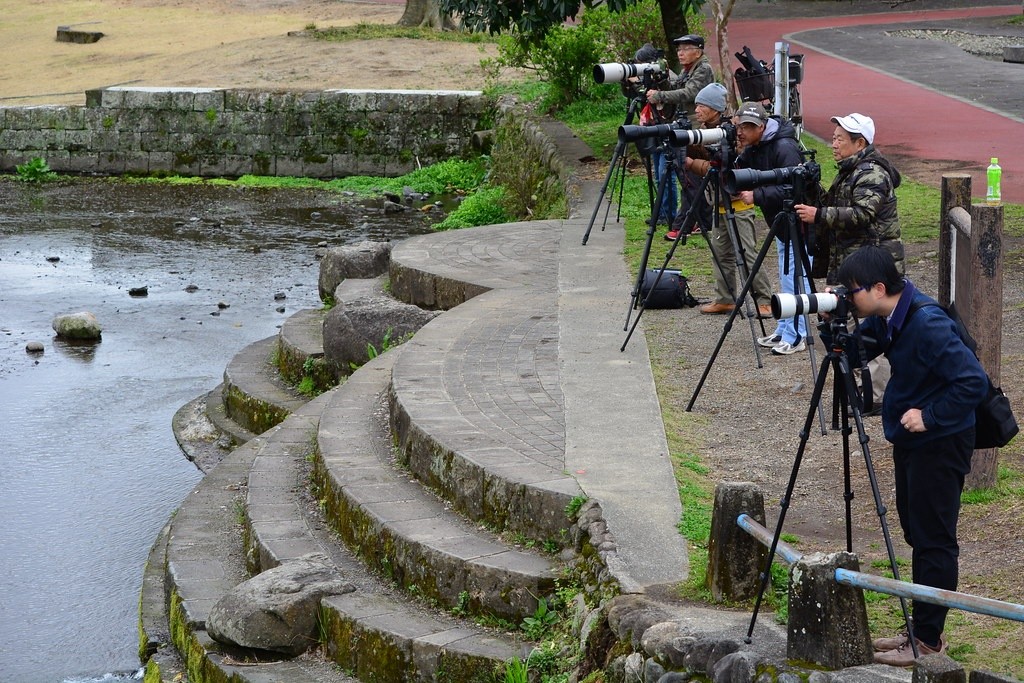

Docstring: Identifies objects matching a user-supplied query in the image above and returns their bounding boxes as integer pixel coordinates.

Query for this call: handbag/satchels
[972,385,1019,449]
[640,269,686,310]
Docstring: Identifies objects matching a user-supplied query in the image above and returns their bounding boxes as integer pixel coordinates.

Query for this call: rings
[904,424,909,430]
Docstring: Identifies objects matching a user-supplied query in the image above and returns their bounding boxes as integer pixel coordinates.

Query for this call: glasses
[676,47,701,52]
[844,284,869,299]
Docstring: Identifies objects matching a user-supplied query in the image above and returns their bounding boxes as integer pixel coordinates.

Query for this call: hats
[636,43,658,63]
[694,83,727,112]
[674,35,705,49]
[738,101,768,127]
[829,113,875,145]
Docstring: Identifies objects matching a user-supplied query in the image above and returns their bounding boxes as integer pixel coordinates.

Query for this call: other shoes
[644,218,667,225]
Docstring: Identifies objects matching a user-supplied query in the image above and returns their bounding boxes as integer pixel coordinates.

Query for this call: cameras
[772,287,854,320]
[594,58,670,84]
[670,123,737,148]
[618,111,692,143]
[726,149,820,194]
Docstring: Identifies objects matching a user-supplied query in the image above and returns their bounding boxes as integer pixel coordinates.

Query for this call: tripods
[582,80,923,663]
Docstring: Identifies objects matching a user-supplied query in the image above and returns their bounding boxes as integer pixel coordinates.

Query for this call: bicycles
[736,55,805,142]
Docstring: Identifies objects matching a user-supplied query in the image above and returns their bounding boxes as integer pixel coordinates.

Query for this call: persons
[684,82,774,318]
[792,112,905,418]
[621,35,712,241]
[728,101,809,354]
[820,244,990,667]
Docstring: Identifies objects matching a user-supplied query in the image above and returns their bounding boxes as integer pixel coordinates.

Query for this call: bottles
[986,157,1001,203]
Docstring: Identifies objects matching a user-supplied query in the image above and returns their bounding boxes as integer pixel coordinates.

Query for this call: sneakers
[700,302,739,314]
[664,229,691,241]
[691,223,703,234]
[872,629,949,652]
[757,334,781,347]
[755,304,772,317]
[771,340,805,355]
[874,636,945,667]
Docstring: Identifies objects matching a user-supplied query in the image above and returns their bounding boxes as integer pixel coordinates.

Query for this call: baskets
[736,73,775,102]
[771,53,804,84]
